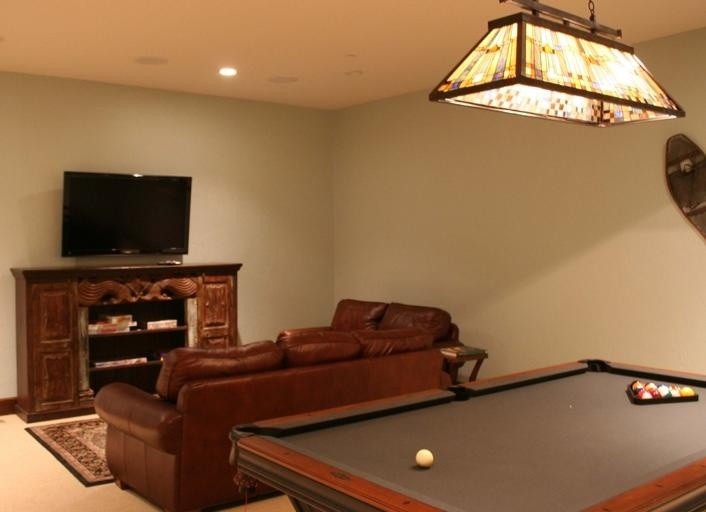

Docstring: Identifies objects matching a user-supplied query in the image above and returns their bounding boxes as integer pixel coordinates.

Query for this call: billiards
[415,450,434,467]
[630,378,696,399]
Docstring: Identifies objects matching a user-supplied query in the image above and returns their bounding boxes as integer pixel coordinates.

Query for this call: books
[88,313,138,335]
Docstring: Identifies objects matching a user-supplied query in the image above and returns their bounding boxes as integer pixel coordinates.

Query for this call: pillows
[332,299,388,326]
[157,340,283,402]
[276,326,361,367]
[376,302,451,344]
[348,327,434,359]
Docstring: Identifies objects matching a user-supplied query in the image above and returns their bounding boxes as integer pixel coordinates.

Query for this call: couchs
[94,323,461,512]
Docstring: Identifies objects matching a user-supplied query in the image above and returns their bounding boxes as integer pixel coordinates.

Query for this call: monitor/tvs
[62,171,192,257]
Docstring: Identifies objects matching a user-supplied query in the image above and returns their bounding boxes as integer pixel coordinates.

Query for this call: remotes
[157,260,181,265]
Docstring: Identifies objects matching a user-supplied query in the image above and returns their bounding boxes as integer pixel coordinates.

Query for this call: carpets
[24,417,115,487]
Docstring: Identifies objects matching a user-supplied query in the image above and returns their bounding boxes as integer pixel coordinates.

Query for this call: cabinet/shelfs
[9,263,243,423]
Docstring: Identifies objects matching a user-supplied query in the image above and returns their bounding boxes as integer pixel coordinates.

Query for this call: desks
[230,359,705,511]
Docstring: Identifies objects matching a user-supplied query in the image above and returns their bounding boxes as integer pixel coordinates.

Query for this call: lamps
[428,1,686,129]
